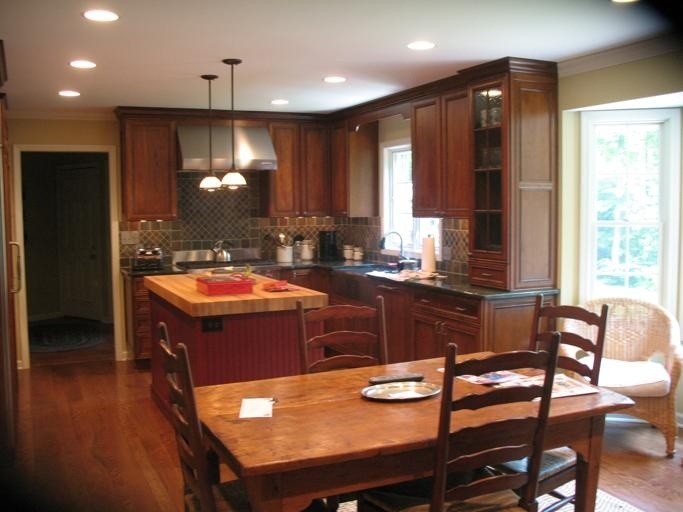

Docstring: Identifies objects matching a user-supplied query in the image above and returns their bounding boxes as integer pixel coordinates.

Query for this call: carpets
[28,321,112,353]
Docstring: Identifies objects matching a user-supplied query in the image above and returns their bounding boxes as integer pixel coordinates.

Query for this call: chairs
[562,293,681,460]
[358,327,563,511]
[291,294,391,511]
[149,322,326,512]
[485,291,607,512]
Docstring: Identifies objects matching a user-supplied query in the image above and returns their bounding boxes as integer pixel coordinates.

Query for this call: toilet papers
[421,235,438,274]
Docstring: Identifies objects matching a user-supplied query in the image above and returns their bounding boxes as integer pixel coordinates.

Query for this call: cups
[276,241,314,263]
[341,245,364,260]
[478,107,501,168]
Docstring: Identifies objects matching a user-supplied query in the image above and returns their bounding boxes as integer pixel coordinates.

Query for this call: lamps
[199,74,221,192]
[221,58,248,189]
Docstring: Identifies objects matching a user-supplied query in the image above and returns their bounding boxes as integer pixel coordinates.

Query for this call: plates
[362,381,441,401]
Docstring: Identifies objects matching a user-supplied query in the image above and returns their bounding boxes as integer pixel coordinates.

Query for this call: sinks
[330,262,399,275]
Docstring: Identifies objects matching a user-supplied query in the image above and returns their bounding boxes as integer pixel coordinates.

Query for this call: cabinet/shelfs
[116,104,178,222]
[330,110,379,217]
[258,111,330,218]
[401,287,556,363]
[462,56,559,290]
[410,72,469,219]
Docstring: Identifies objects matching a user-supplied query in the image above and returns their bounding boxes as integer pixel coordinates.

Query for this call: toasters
[131,246,164,270]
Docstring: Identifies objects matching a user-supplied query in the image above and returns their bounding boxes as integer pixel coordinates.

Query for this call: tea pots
[209,240,236,263]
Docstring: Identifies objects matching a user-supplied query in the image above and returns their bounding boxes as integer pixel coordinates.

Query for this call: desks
[190,349,631,512]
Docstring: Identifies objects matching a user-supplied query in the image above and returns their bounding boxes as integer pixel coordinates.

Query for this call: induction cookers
[172,249,277,275]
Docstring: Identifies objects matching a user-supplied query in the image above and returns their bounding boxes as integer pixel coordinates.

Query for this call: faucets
[378,231,404,263]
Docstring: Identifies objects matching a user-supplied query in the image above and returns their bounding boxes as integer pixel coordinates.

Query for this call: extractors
[176,125,277,173]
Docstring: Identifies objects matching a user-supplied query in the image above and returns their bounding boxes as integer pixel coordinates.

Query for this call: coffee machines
[317,229,338,262]
